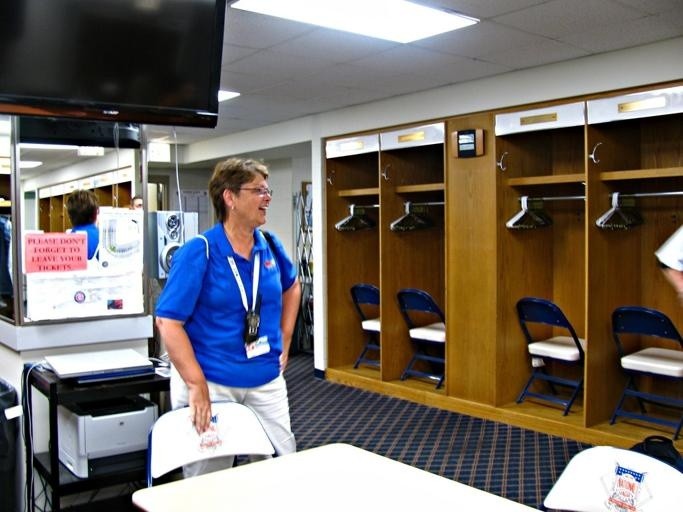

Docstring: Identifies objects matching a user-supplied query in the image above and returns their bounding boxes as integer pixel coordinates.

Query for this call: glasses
[236,187,273,196]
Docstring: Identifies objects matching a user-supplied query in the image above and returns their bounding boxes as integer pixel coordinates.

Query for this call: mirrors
[17,143,138,271]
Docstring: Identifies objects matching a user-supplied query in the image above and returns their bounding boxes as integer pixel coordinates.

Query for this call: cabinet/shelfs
[495,79,683,456]
[323,118,448,411]
[19,353,170,512]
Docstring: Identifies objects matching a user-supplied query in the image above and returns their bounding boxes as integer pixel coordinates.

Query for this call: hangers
[334,191,434,232]
[503,192,635,233]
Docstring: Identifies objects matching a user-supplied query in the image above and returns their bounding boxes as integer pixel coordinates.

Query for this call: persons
[67,188,97,263]
[153,158,302,480]
[654,222,683,299]
[127,195,144,211]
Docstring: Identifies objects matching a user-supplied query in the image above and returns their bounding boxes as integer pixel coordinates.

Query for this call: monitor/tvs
[1,0,227,129]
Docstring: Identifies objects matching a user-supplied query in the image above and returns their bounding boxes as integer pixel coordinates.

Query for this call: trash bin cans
[0,377,23,512]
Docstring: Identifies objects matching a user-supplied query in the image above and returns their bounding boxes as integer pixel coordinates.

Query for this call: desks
[130,442,544,512]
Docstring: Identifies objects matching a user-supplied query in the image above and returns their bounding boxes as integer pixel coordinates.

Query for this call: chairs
[349,279,380,375]
[146,402,277,491]
[513,296,584,415]
[611,304,683,441]
[395,289,444,390]
[538,444,683,511]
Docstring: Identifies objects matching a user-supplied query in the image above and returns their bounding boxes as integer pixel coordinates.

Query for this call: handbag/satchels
[629,433,681,466]
